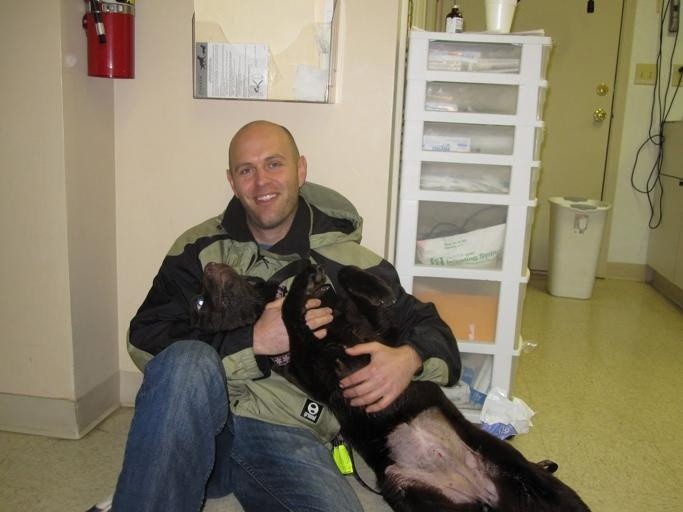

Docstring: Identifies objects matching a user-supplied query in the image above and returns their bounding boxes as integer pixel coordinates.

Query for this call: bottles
[445,4,464,34]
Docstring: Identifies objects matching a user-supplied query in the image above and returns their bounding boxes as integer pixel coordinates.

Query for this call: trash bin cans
[548,196,612,299]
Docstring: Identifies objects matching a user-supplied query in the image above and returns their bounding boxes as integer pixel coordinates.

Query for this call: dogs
[189,261,592,512]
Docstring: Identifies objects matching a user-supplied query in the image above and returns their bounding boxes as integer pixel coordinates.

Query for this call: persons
[110,119,463,511]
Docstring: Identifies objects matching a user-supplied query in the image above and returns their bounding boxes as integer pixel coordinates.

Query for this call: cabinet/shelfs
[388,28,558,426]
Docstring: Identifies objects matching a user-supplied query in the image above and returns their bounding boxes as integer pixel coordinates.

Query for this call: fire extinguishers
[84,1,136,77]
